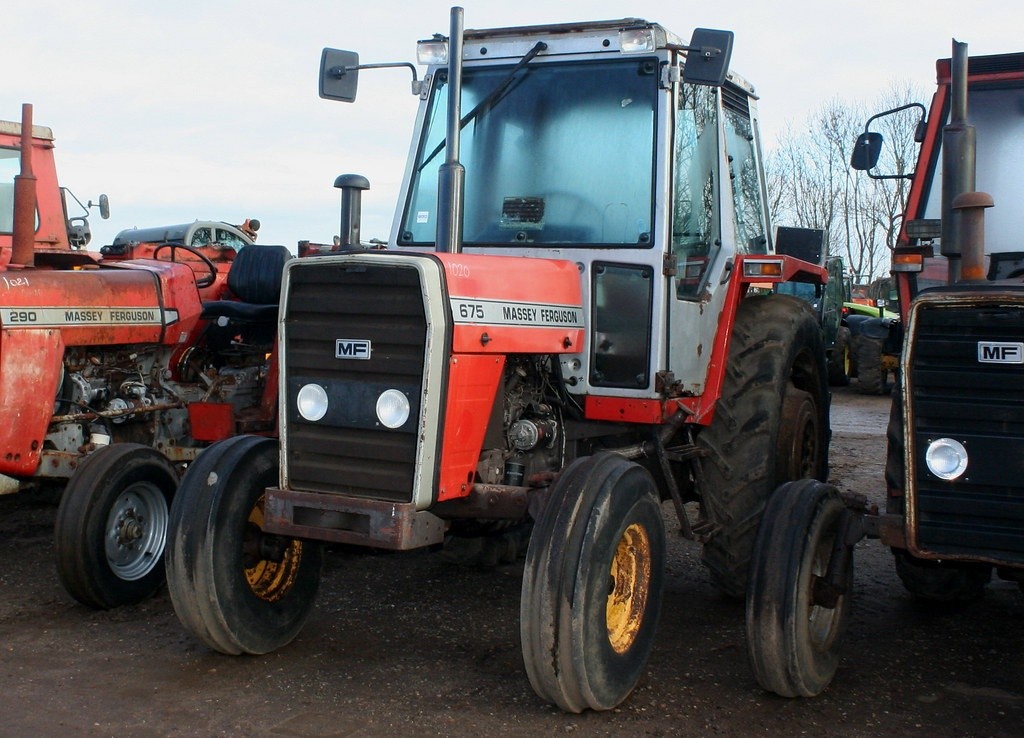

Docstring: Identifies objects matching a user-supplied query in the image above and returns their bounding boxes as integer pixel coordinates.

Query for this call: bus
[162,6,833,715]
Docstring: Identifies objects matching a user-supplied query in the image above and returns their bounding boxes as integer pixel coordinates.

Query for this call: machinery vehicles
[746,36,1022,701]
[771,255,866,386]
[0,101,307,611]
[856,291,907,395]
